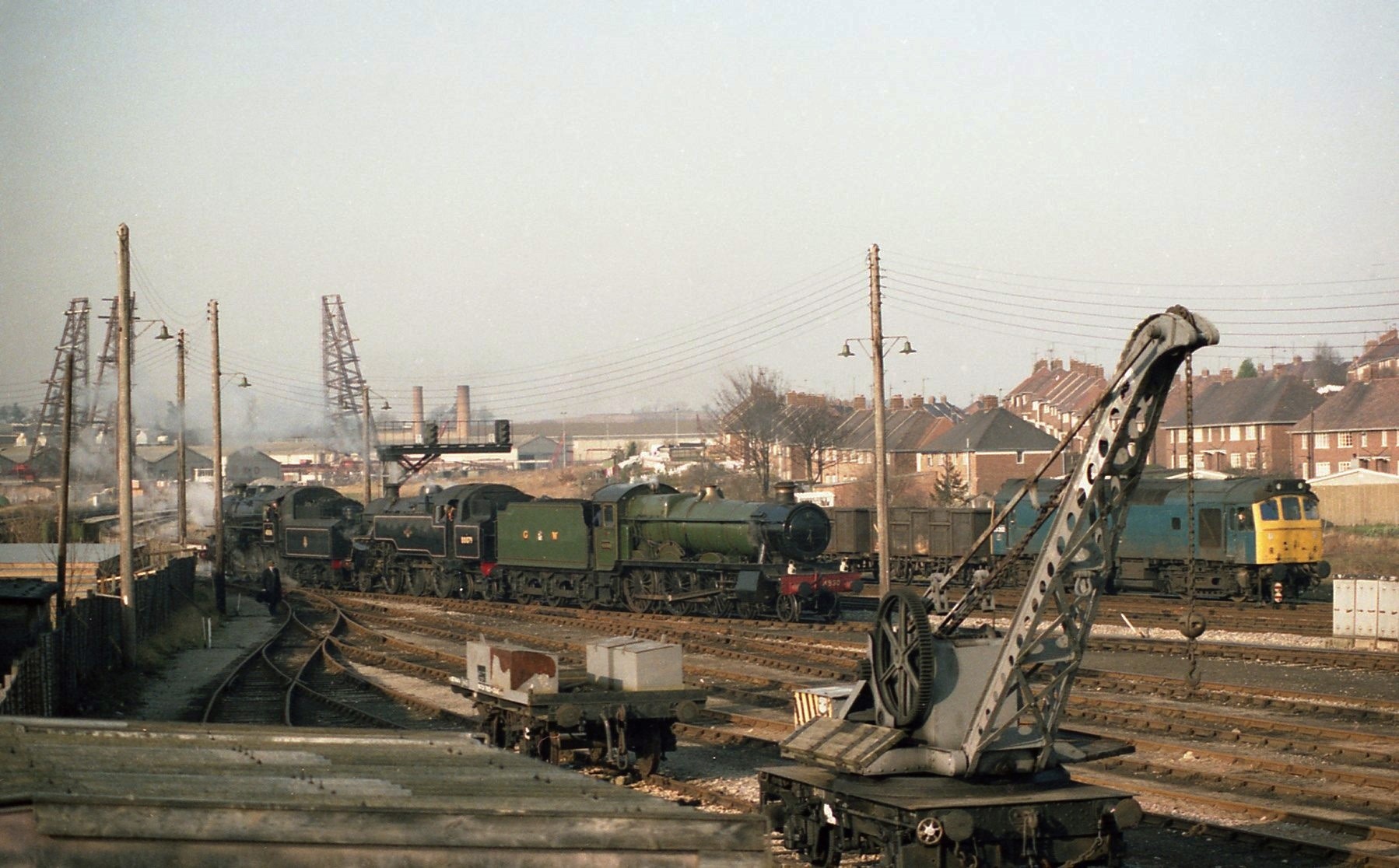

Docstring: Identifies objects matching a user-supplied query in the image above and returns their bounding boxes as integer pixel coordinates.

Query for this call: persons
[261,560,283,616]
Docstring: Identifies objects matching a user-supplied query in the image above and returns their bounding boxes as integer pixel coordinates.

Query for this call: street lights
[836,335,918,599]
[115,317,177,671]
[212,370,252,614]
[362,385,392,506]
[560,412,567,468]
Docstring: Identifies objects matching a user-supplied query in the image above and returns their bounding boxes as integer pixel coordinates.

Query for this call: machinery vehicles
[753,302,1218,867]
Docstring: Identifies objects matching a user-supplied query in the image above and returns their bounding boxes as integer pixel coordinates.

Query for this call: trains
[198,478,867,624]
[813,467,1332,610]
